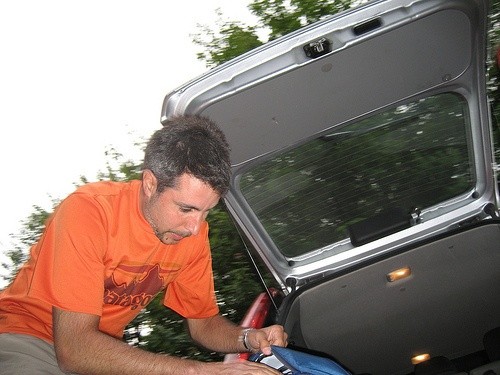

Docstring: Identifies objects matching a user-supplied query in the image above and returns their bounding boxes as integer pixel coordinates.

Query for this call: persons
[0,114,288,375]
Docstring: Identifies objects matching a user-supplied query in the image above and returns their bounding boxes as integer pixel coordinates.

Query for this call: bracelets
[240,327,255,351]
